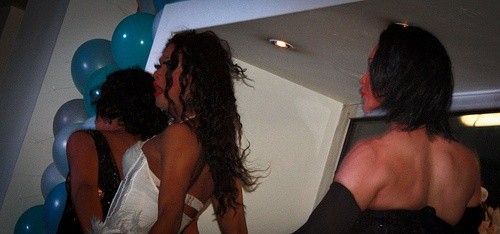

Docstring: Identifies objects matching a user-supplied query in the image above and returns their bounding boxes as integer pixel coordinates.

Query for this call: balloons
[13,0,179,234]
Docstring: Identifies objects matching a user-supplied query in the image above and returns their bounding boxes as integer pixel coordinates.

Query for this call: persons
[56,28,271,234]
[290,23,485,234]
